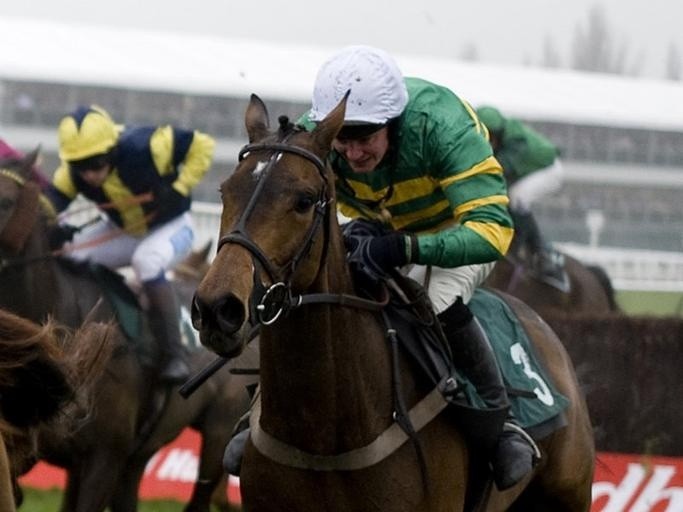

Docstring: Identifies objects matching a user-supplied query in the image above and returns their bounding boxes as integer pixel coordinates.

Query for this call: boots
[433,315,535,492]
[146,282,188,384]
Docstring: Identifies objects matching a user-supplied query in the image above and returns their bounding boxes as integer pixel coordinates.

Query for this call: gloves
[342,217,404,301]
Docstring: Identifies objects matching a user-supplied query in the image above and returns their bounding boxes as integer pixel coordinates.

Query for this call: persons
[293,46,541,493]
[476,105,566,276]
[42,103,216,386]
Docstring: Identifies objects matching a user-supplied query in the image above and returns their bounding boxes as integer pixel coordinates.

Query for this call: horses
[192,89,599,508]
[0,139,258,506]
[493,225,681,457]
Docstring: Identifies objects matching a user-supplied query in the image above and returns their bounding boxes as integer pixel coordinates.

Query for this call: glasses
[74,155,106,170]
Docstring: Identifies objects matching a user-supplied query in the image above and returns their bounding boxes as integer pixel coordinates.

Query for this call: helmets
[307,48,409,140]
[56,106,118,162]
